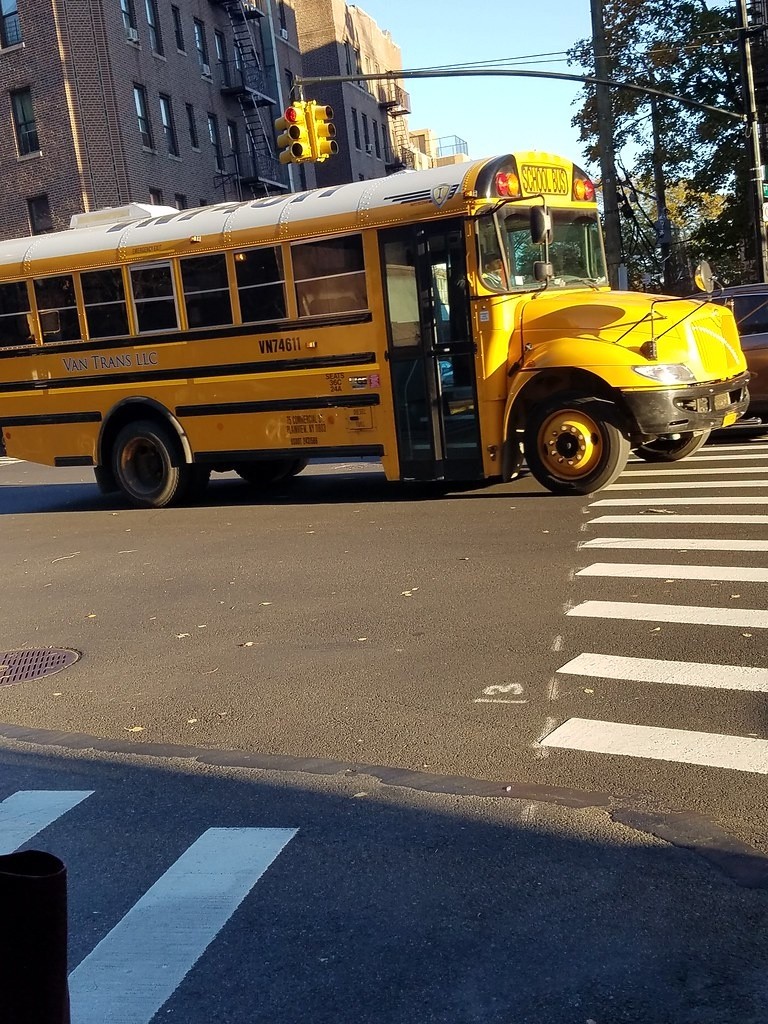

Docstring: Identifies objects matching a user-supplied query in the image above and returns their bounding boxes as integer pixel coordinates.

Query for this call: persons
[61,262,285,342]
[453,234,508,290]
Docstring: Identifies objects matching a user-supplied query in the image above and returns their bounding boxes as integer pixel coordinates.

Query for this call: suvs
[684,283,768,431]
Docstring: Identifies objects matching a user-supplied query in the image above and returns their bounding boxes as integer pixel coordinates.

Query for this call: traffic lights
[274,106,312,165]
[305,100,339,163]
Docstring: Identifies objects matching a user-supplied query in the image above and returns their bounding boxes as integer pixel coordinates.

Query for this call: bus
[0,153,750,510]
